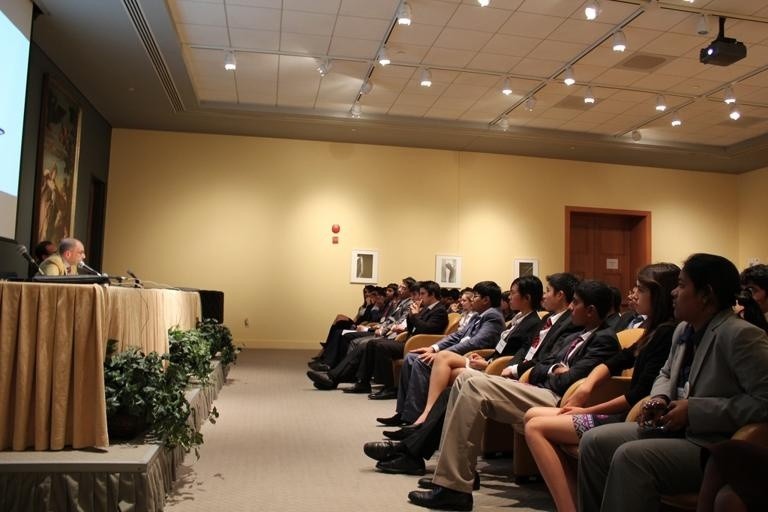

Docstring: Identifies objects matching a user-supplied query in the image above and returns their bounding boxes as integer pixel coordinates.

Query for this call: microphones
[77,261,101,276]
[127,269,139,281]
[16,245,45,276]
[102,273,125,279]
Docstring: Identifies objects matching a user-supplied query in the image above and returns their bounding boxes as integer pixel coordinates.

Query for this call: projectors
[700,40,746,66]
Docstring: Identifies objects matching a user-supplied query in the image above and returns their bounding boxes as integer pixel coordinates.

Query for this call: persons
[35,240,58,262]
[34,238,86,277]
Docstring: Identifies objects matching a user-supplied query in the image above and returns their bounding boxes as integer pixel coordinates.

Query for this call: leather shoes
[376,455,426,474]
[365,439,405,458]
[408,488,474,512]
[306,341,415,439]
[419,472,481,491]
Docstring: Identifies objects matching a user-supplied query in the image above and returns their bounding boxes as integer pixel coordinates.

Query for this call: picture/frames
[350,249,379,285]
[513,258,539,282]
[435,255,463,288]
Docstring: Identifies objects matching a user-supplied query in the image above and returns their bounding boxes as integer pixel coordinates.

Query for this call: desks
[0,280,224,452]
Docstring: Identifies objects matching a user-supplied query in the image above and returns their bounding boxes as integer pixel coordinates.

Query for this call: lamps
[655,17,741,132]
[317,55,333,80]
[479,1,490,7]
[493,4,644,140]
[224,48,238,72]
[350,1,435,118]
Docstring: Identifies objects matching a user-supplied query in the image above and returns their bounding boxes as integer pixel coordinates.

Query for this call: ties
[560,335,581,363]
[522,319,550,362]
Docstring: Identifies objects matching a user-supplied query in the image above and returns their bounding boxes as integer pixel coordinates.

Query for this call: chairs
[389,305,768,512]
